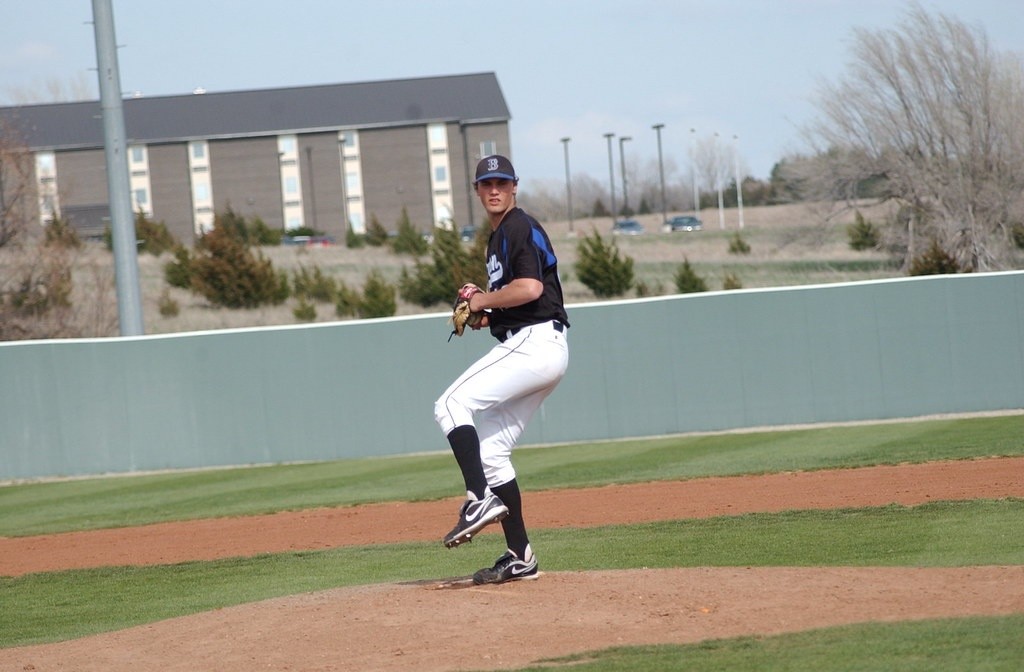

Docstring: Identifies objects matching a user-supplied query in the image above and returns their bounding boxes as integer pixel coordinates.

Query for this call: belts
[502,319,563,333]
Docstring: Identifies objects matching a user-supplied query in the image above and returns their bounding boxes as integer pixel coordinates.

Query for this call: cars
[611,219,649,237]
[461,227,483,240]
[662,215,703,233]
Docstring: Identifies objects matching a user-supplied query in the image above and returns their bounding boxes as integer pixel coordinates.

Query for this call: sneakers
[443,496,509,549]
[472,549,539,585]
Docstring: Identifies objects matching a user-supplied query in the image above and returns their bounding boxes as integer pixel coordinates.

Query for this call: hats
[475,155,515,183]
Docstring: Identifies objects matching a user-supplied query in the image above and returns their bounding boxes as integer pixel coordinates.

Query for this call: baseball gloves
[446,282,487,343]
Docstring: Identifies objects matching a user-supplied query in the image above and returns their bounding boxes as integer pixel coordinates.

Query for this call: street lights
[560,136,576,230]
[653,123,673,221]
[603,133,620,222]
[620,136,633,218]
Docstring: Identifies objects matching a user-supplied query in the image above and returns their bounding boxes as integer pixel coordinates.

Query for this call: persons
[434,155,571,589]
